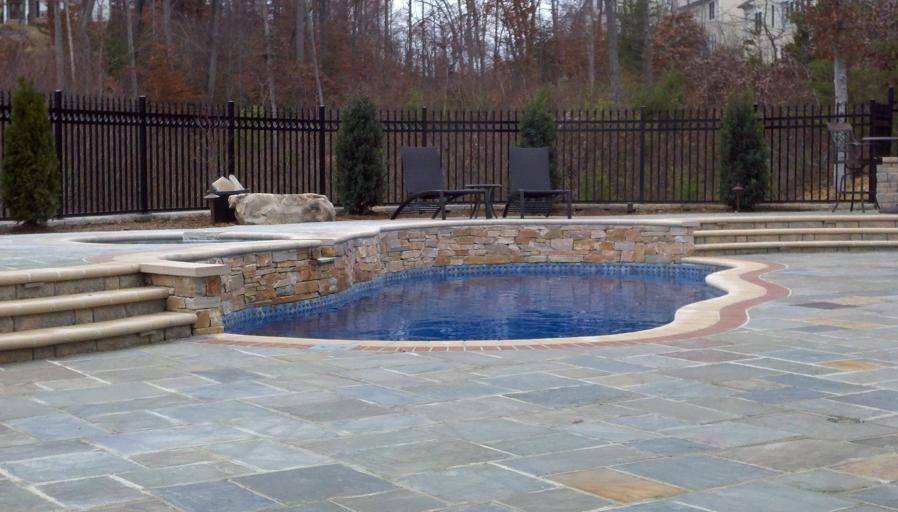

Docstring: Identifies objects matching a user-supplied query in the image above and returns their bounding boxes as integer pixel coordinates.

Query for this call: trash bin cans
[204,189,250,222]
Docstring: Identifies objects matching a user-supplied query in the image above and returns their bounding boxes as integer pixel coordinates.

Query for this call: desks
[465,184,502,218]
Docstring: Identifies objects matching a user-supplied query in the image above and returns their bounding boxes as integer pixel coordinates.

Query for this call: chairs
[503,144,573,219]
[390,146,487,220]
[827,122,881,213]
